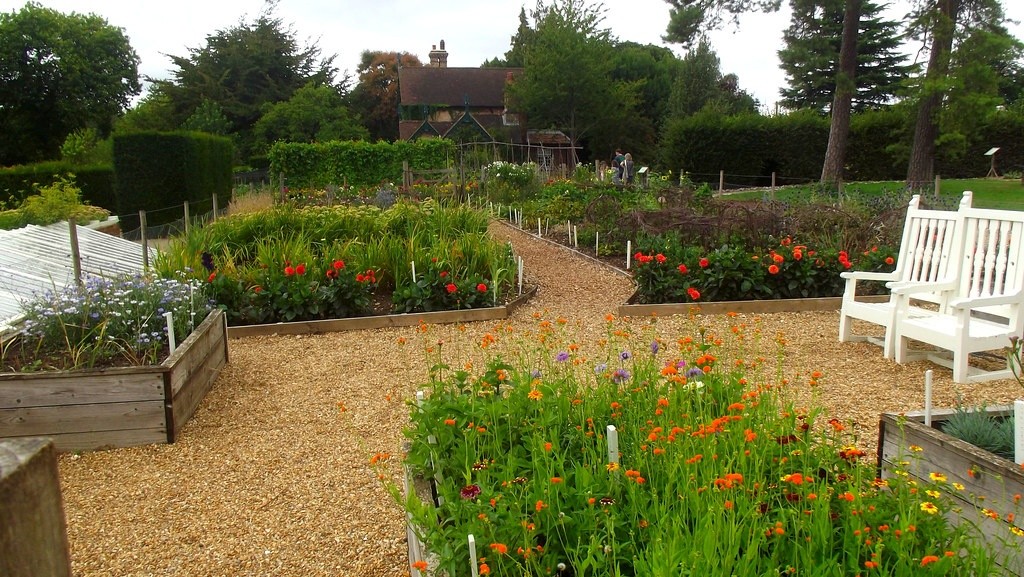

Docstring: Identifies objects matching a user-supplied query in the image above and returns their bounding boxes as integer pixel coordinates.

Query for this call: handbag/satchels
[622,160,628,179]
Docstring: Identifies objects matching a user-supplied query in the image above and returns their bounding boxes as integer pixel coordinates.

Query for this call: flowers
[0,263,215,372]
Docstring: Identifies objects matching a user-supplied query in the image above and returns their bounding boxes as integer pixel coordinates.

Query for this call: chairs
[837,194,958,358]
[891,191,1024,384]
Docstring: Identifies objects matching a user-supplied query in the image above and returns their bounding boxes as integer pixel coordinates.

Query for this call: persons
[599,148,635,184]
